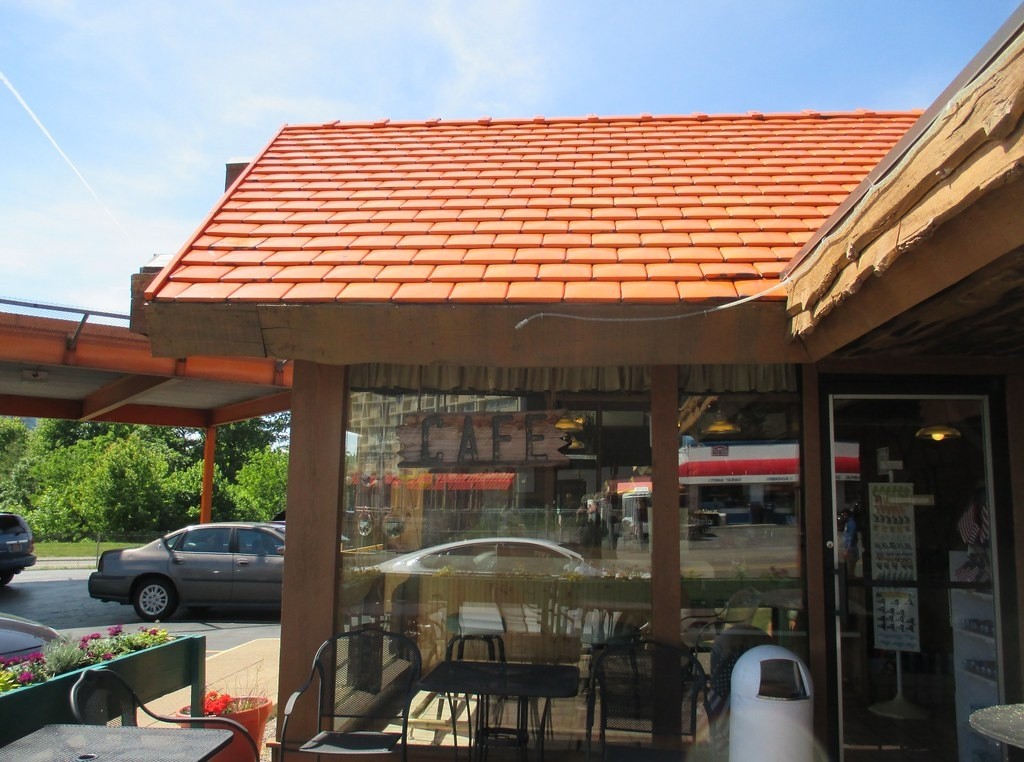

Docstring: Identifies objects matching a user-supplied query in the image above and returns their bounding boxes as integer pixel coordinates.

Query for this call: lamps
[555,409,584,433]
[701,397,741,435]
[916,426,961,440]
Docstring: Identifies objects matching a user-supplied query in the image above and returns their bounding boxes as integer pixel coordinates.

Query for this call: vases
[175,696,272,762]
[342,573,802,607]
[0,634,197,748]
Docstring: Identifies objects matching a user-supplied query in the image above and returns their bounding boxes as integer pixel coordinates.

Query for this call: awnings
[353,472,517,493]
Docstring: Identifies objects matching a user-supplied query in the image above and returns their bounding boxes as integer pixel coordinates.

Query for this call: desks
[0,723,234,762]
[970,703,1024,750]
[416,660,580,762]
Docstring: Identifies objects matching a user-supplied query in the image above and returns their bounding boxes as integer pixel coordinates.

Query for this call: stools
[435,613,506,743]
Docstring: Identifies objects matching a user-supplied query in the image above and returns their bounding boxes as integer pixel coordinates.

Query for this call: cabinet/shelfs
[943,540,1003,762]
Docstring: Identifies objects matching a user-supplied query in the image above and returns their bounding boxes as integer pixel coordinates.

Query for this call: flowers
[182,691,262,715]
[0,623,176,693]
[433,563,790,596]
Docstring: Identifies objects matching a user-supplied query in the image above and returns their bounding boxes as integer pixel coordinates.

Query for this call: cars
[349,537,725,667]
[0,612,68,667]
[88,506,286,623]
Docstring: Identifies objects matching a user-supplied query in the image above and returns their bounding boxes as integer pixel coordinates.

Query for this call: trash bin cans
[728,645,815,761]
[709,624,778,761]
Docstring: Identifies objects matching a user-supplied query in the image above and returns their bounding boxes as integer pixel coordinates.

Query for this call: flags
[956,505,989,546]
[955,560,981,582]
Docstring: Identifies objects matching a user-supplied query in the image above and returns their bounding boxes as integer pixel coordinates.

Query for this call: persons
[577,492,619,560]
[840,509,859,579]
[633,499,648,550]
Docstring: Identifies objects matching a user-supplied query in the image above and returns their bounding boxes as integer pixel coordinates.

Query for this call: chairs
[279,627,423,762]
[586,626,778,762]
[71,669,261,762]
[682,586,758,690]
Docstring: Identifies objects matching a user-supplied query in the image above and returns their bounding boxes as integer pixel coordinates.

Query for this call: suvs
[0,512,36,586]
